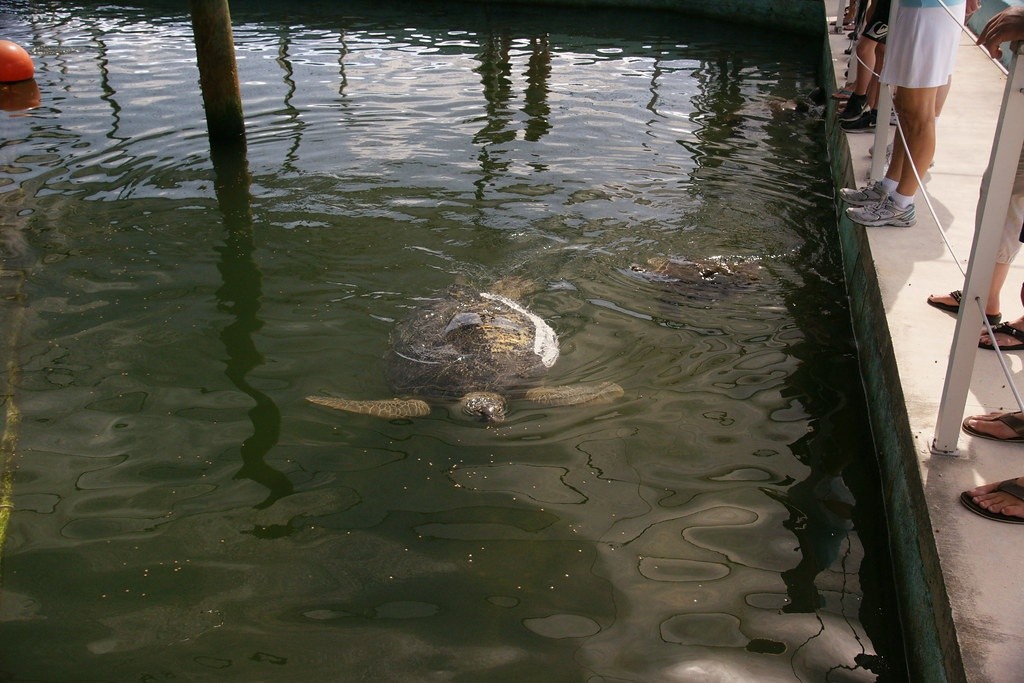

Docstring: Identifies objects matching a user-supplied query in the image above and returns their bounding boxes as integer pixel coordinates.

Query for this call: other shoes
[890,107,899,125]
[830,14,864,39]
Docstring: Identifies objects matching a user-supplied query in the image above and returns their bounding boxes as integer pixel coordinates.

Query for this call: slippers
[978,322,1024,351]
[962,411,1024,442]
[960,477,1024,525]
[830,89,854,101]
[927,290,1002,325]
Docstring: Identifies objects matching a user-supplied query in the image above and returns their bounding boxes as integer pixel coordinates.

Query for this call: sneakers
[845,194,917,227]
[839,179,889,206]
[839,109,878,134]
[839,92,870,121]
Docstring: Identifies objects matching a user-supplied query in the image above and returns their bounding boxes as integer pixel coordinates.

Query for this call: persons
[959,225,1024,525]
[826,0,981,228]
[927,0,1023,351]
